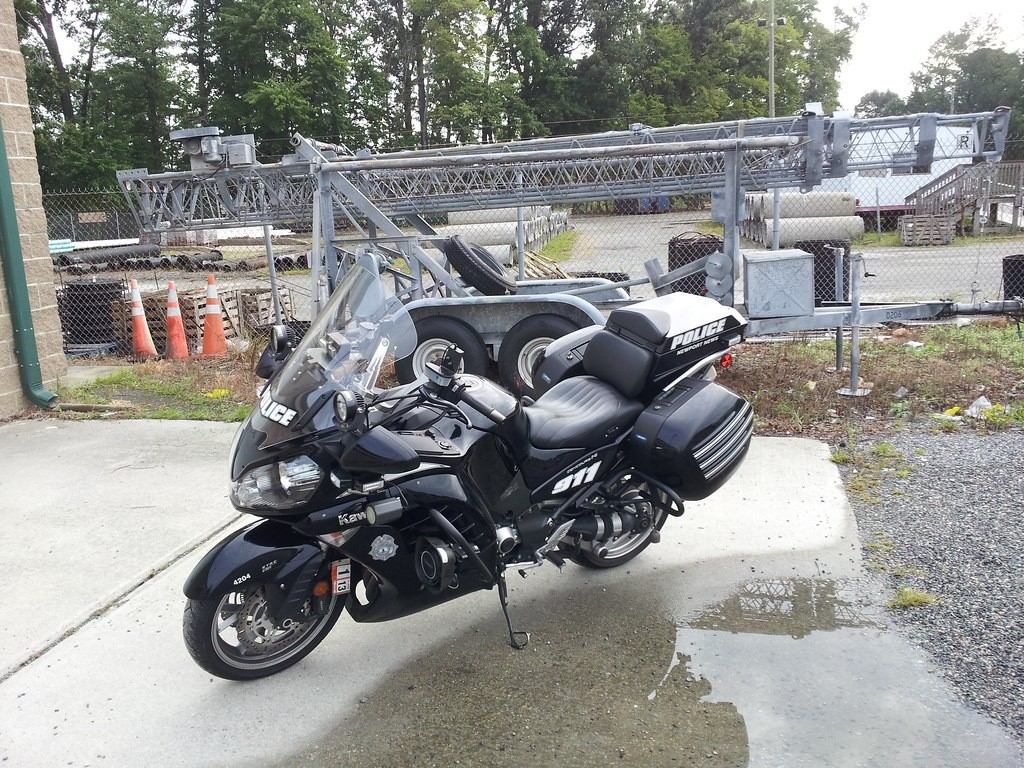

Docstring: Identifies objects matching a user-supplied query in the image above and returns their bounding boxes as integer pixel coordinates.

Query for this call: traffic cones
[130,279,164,362]
[161,281,191,361]
[193,274,232,360]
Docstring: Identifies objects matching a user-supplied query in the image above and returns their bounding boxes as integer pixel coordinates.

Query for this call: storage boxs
[743,248,815,319]
[111,287,245,358]
[792,239,850,301]
[240,284,296,331]
[668,231,724,297]
[897,214,957,247]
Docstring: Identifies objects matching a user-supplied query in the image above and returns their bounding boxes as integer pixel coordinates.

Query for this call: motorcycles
[184,265,753,683]
